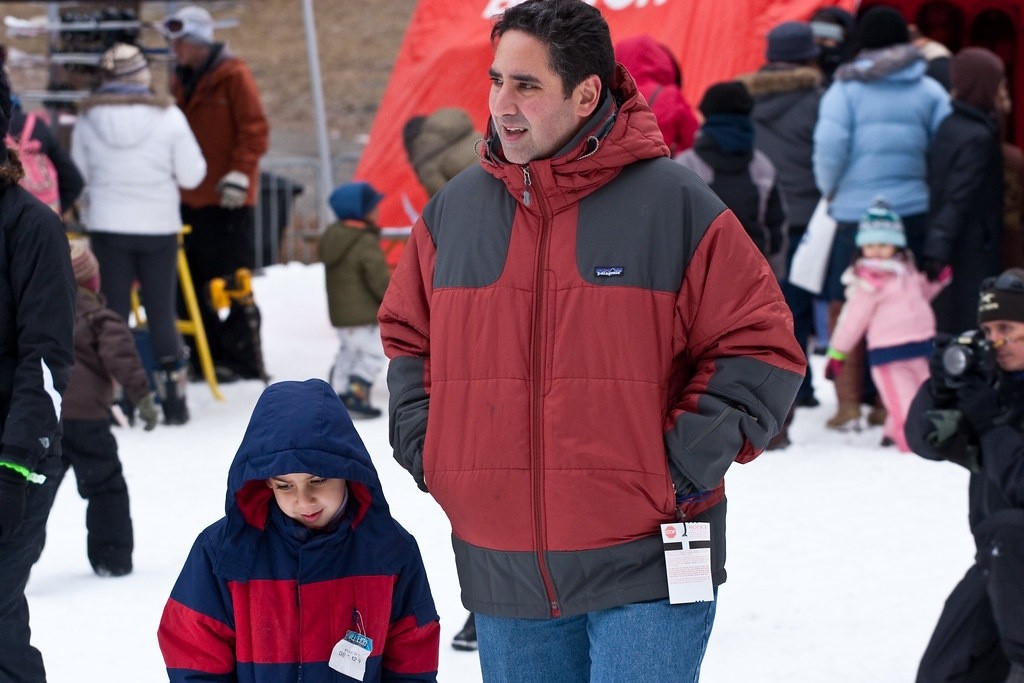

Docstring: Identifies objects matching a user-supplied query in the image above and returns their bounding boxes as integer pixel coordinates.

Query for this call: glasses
[161,10,192,37]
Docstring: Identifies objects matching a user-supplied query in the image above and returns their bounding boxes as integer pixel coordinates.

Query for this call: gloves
[138,395,158,432]
[931,329,1001,435]
[215,181,249,209]
[0,474,26,544]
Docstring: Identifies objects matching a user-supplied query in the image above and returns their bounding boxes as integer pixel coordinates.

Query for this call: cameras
[943,329,994,376]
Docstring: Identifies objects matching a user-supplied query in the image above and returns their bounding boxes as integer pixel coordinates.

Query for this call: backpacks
[2,113,59,218]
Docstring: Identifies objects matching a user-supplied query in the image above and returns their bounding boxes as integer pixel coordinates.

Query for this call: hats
[328,185,383,219]
[977,267,1024,322]
[858,6,912,53]
[169,8,215,44]
[853,203,909,249]
[812,10,844,42]
[98,44,153,89]
[696,82,752,118]
[70,242,101,295]
[765,22,822,60]
[950,48,1004,110]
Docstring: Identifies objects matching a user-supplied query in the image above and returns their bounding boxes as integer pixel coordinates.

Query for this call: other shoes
[766,434,791,449]
[451,612,482,651]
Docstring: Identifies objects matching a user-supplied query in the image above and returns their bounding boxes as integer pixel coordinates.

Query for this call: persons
[0,44,78,683]
[616,0,1024,450]
[2,98,84,217]
[378,0,805,683]
[905,269,1024,683]
[315,182,392,418]
[38,241,148,578]
[402,108,484,648]
[70,43,207,427]
[165,4,271,380]
[157,379,442,683]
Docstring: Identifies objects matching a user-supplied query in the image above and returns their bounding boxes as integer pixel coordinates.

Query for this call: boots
[158,364,190,425]
[824,302,859,434]
[345,374,381,417]
[870,394,888,426]
